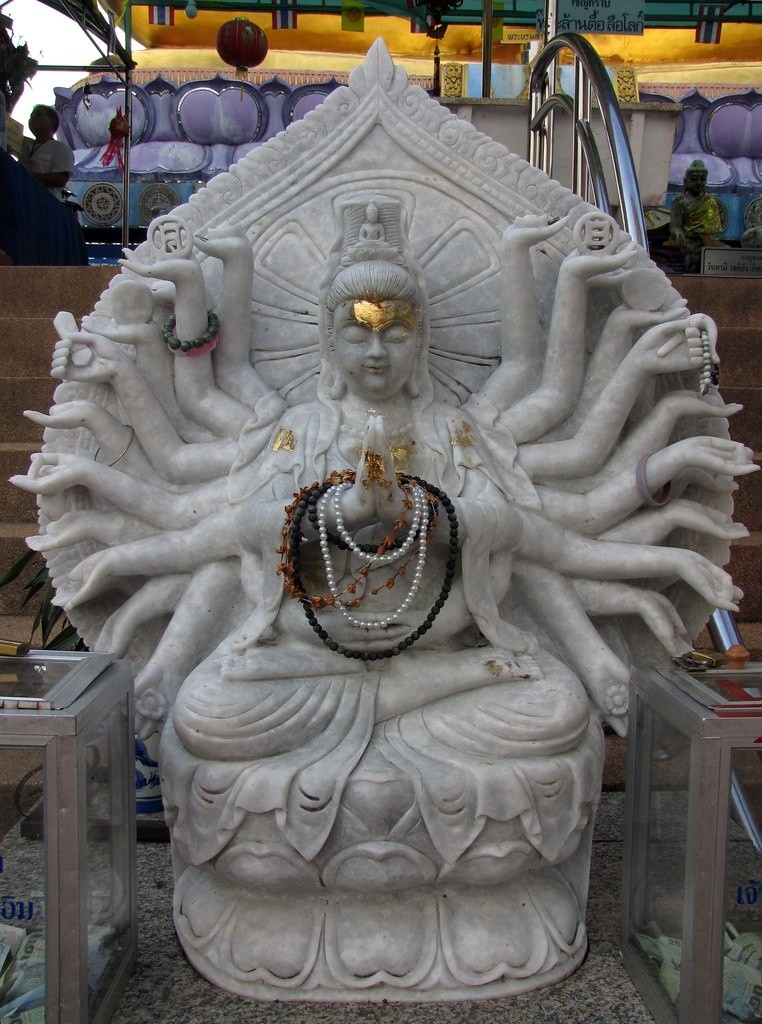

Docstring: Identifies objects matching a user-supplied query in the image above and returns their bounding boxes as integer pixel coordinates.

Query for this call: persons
[22,105,74,202]
[663,159,733,253]
[8,200,761,761]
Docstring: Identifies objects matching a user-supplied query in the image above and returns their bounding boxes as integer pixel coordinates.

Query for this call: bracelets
[162,308,220,358]
[94,424,135,467]
[699,330,722,397]
[636,453,672,507]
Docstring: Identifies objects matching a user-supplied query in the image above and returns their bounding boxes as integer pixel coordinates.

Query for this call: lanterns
[216,17,268,100]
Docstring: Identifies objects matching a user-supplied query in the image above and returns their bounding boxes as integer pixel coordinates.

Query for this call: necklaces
[274,469,459,662]
